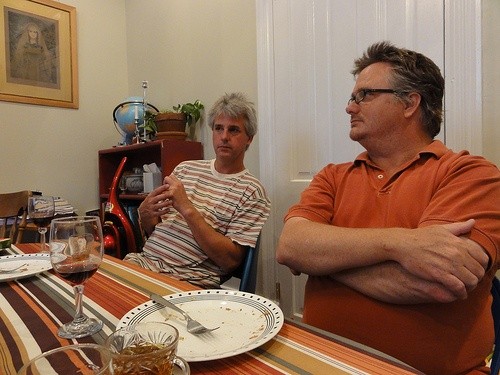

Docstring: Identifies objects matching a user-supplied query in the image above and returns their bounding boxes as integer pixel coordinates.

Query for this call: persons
[122,91,272,289]
[276,39,500,375]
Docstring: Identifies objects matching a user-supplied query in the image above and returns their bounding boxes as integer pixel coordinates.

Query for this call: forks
[149,293,220,334]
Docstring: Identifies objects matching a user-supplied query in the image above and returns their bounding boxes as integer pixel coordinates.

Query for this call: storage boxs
[143,172,161,193]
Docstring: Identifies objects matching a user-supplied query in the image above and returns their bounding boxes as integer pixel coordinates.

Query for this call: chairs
[233,233,262,294]
[0,191,34,245]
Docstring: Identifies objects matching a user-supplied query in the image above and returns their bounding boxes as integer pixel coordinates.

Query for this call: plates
[0,253,67,282]
[116,289,285,361]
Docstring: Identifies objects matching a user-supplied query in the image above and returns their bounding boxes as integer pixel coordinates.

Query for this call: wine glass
[50,216,103,338]
[28,197,55,253]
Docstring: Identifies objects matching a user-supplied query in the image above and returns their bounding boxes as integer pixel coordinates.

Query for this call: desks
[0,239,423,375]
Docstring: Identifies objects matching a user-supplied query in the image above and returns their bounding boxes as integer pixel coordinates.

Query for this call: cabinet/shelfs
[98,141,205,258]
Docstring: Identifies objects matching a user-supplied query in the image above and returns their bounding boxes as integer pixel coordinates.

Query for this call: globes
[113,95,152,145]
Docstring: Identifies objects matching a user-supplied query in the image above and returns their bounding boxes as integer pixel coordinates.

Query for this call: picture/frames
[0,0,80,110]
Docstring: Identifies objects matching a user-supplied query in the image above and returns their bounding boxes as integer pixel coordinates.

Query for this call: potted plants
[138,99,206,140]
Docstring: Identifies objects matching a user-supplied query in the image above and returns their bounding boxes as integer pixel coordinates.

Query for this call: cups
[105,322,179,375]
[18,344,114,375]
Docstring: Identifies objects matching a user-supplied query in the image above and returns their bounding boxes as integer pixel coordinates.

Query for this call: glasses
[348,89,394,105]
